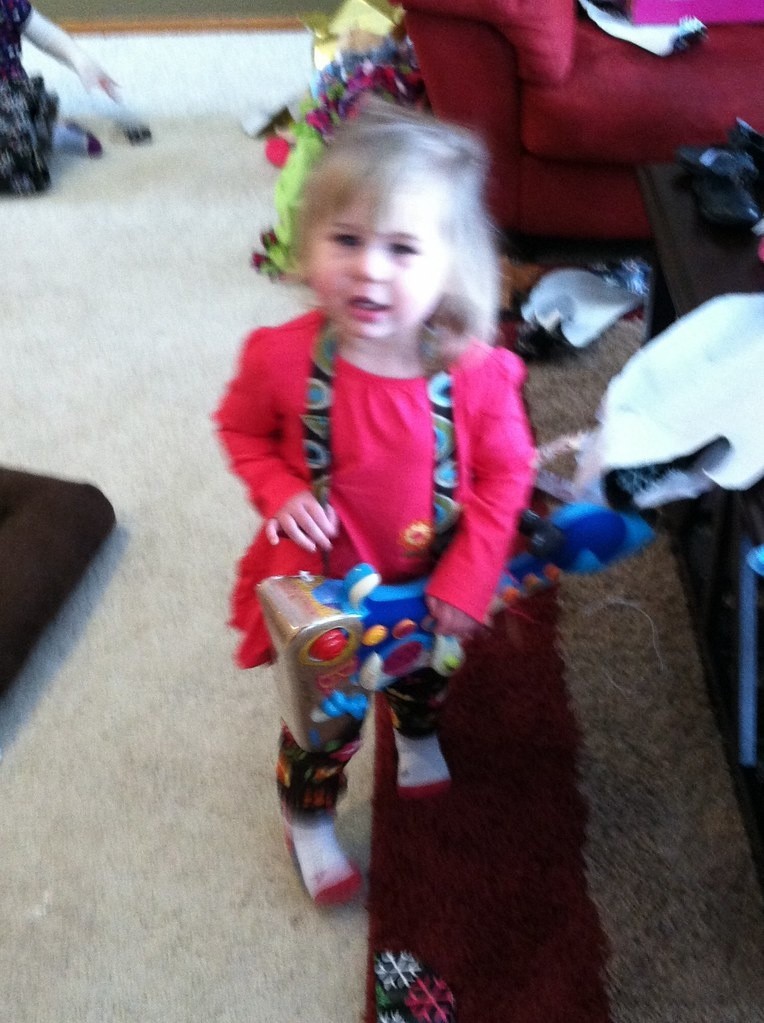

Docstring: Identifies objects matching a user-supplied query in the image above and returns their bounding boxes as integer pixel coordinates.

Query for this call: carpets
[357,308,763,1023]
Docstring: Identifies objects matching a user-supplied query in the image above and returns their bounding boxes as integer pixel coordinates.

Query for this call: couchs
[390,1,763,246]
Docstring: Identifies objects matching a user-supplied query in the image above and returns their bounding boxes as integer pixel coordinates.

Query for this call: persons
[214,124,535,905]
[0,1,121,196]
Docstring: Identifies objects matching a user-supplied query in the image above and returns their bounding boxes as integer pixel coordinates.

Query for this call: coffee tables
[631,161,764,766]
